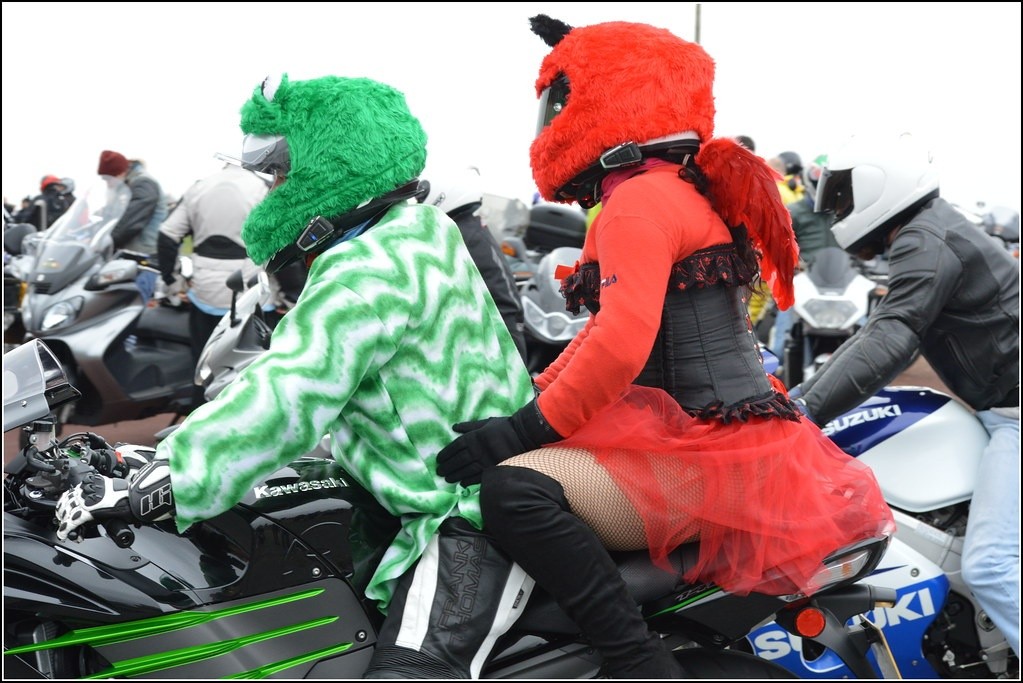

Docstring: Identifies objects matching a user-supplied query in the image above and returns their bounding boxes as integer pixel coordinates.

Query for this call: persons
[22,175,75,231]
[790,126,1020,658]
[735,136,754,153]
[412,160,528,368]
[54,75,540,679]
[15,198,32,221]
[156,132,291,414]
[435,13,898,678]
[92,150,168,255]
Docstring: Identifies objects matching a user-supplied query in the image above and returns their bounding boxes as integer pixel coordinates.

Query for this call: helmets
[813,135,940,257]
[531,71,698,201]
[408,162,483,221]
[781,152,804,175]
[242,128,291,192]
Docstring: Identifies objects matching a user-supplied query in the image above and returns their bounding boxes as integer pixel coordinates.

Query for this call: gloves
[54,459,174,541]
[431,395,554,487]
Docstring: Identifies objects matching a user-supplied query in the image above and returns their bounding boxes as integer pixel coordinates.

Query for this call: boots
[478,459,685,677]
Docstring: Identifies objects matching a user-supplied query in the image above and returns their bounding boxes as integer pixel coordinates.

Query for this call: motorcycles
[4,175,1020,679]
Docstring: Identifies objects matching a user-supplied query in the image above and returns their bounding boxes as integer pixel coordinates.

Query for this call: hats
[98,152,126,176]
